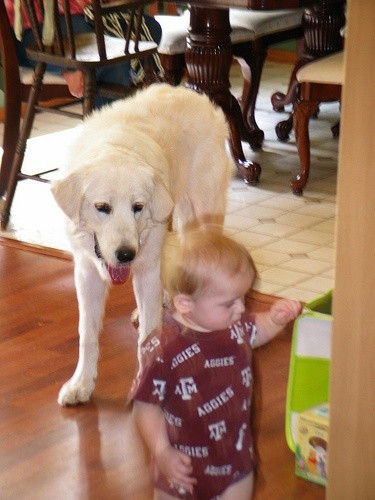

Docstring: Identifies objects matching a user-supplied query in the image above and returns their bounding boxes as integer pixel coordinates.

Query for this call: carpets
[0,60,337,311]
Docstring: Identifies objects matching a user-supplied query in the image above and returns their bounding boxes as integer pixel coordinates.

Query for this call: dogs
[50,83,233,407]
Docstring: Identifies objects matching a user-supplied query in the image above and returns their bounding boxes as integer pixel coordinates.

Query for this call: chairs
[0,0,160,230]
[290,50,345,195]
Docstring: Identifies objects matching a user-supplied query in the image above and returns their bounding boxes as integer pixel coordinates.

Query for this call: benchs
[134,0,310,142]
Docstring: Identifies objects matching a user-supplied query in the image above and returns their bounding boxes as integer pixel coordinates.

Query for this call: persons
[124,229,302,500]
[3,0,162,114]
